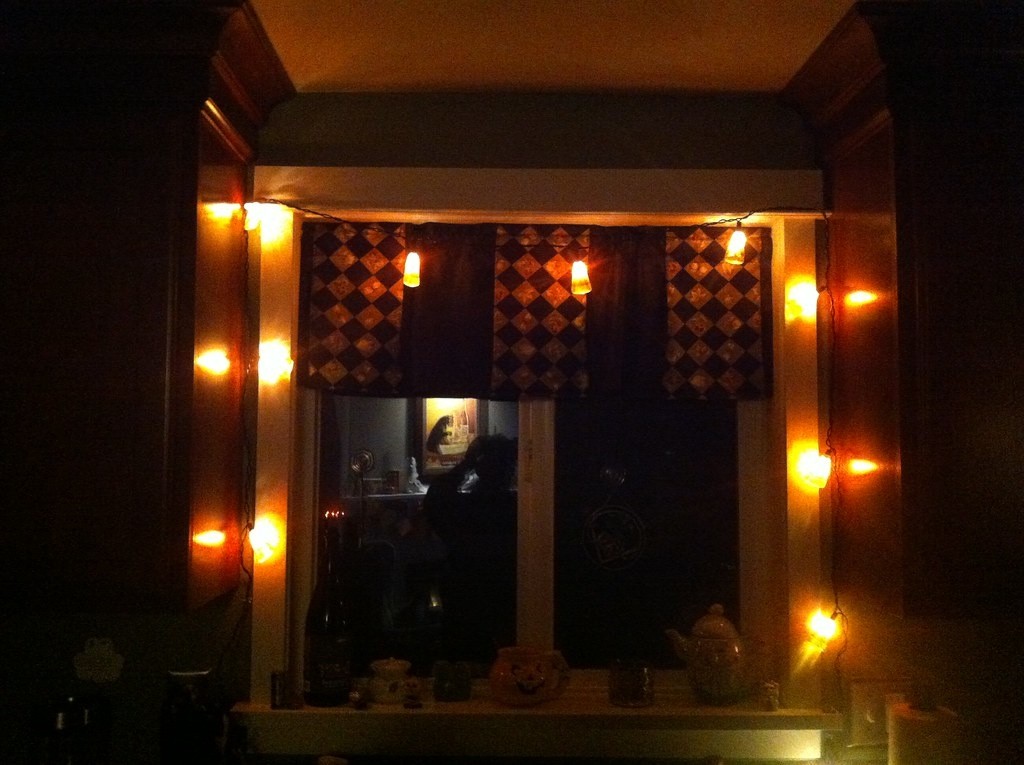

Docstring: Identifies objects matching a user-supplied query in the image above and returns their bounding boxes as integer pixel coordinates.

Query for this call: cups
[606,657,656,709]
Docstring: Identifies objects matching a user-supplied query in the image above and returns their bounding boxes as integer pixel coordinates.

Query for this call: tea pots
[665,605,746,695]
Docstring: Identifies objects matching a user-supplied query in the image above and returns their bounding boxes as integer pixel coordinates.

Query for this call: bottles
[304,572,350,706]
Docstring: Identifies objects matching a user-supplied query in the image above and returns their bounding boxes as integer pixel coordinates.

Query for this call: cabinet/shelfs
[0,1,301,613]
[784,0,1022,623]
